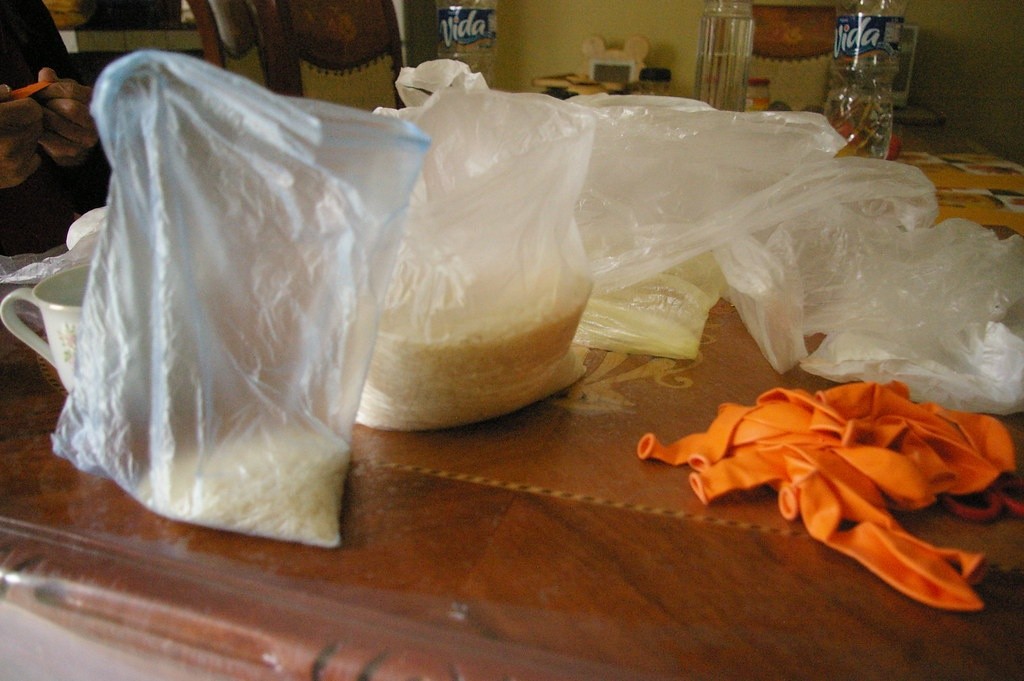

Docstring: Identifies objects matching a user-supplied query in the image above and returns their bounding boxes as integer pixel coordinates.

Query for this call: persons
[0,1,112,259]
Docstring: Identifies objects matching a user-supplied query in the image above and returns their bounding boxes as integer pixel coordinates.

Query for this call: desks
[0,94,1024,681]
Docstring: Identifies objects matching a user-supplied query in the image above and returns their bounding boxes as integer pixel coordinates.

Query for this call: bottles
[824,0,908,161]
[745,78,771,112]
[638,67,672,95]
[696,0,756,113]
[435,0,498,90]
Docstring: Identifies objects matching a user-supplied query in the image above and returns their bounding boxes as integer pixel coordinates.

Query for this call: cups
[0,264,92,393]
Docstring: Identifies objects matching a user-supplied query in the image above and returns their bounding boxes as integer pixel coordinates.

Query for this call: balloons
[636,379,1017,612]
